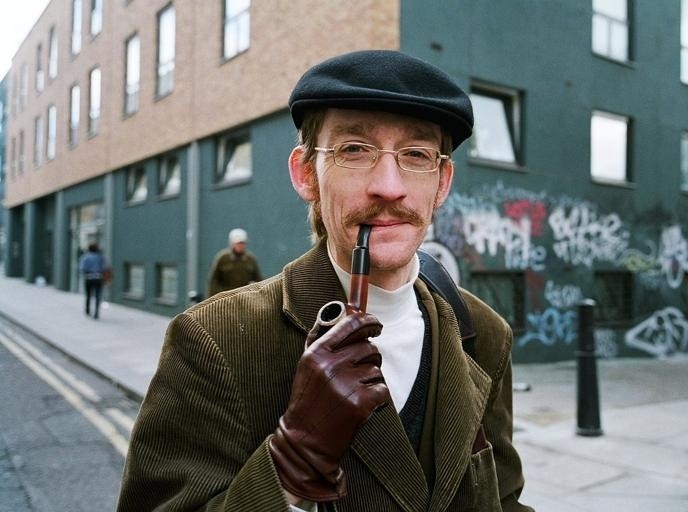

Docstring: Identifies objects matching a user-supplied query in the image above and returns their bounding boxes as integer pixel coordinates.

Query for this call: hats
[229,228,248,244]
[288,49,475,153]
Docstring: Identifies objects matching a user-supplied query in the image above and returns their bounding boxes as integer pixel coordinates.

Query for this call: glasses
[305,142,451,172]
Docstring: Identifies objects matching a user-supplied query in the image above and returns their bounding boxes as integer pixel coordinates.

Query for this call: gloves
[268,313,389,502]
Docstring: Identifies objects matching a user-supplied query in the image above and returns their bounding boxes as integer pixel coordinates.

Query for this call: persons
[111,47,531,511]
[206,226,261,296]
[78,242,104,321]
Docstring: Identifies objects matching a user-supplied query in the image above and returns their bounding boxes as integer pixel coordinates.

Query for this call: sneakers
[85,309,99,319]
